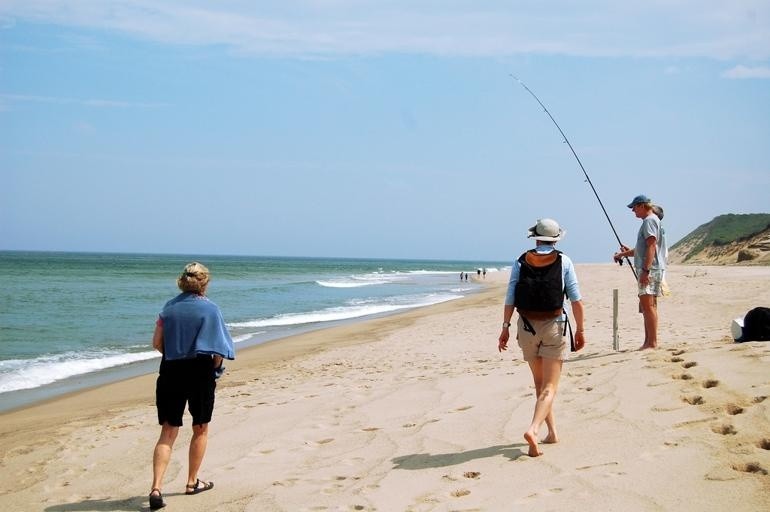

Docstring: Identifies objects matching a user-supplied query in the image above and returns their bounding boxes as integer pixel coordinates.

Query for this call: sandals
[150,488,165,509]
[185,478,214,494]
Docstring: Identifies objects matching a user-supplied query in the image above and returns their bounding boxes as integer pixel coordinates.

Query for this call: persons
[465,273,468,280]
[477,269,481,275]
[611,191,666,354]
[461,271,464,277]
[482,268,486,276]
[144,261,235,511]
[460,273,463,281]
[493,214,585,457]
[645,198,674,348]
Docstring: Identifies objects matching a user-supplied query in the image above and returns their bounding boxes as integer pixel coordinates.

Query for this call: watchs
[502,321,512,328]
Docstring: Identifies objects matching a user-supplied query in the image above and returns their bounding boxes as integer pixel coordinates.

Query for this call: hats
[528,218,565,242]
[628,195,649,208]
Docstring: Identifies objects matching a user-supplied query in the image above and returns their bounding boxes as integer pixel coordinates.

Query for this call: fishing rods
[508,72,640,285]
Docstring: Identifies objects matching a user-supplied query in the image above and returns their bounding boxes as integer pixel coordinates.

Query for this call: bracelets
[577,328,583,332]
[640,266,650,275]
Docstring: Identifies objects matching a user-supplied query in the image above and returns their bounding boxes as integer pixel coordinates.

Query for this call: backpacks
[514,250,562,311]
